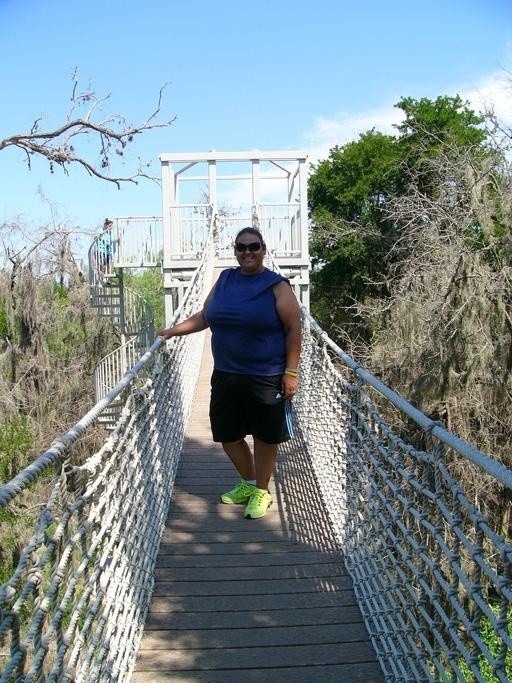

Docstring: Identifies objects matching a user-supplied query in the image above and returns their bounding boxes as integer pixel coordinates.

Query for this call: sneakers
[220,481,257,504]
[244,488,273,519]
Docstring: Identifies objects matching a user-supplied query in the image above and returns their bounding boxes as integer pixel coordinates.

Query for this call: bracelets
[285,368,300,377]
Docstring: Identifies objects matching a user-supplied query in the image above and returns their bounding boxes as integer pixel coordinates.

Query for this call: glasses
[234,242,263,252]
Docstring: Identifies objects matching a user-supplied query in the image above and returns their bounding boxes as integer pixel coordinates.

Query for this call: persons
[155,227,302,520]
[95,218,113,284]
